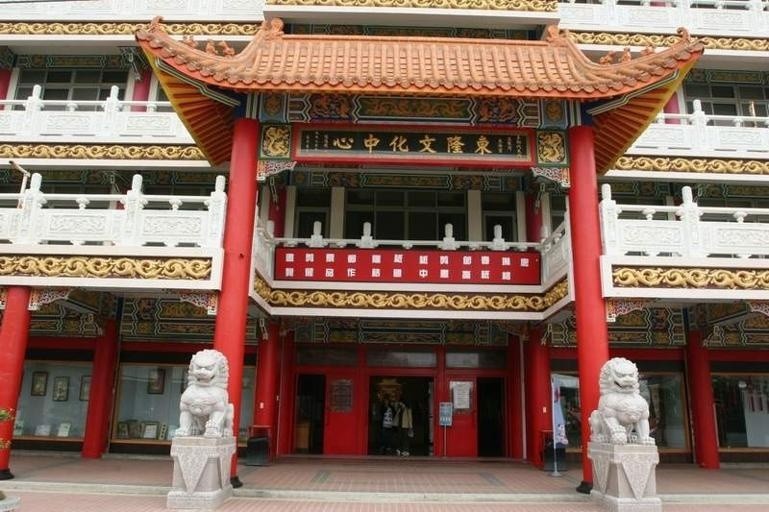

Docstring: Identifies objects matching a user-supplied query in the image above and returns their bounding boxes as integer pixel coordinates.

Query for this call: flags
[551,373,570,446]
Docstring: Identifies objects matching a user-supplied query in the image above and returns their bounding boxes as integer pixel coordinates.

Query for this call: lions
[175,348,235,438]
[588,356,658,445]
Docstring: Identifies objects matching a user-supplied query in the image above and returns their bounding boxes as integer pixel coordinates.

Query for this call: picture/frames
[12,365,171,441]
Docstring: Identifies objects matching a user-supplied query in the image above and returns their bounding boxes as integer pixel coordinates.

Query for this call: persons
[377,394,395,453]
[392,399,415,456]
